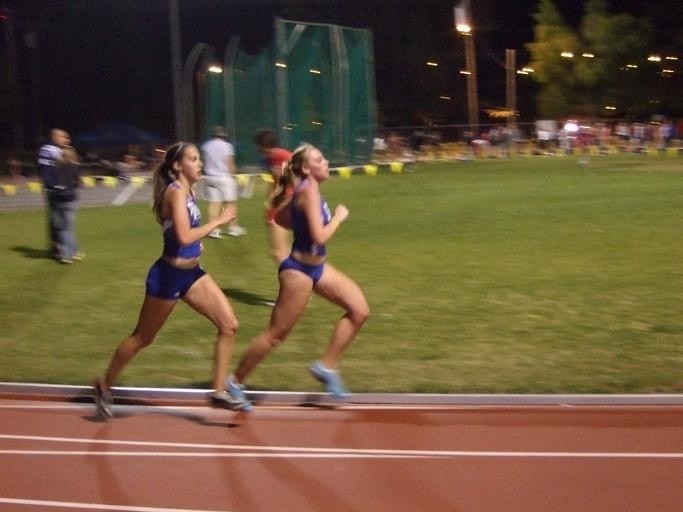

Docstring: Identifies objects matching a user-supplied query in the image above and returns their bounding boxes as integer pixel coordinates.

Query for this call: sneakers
[309,361,349,400]
[69,252,85,260]
[207,392,246,410]
[52,256,72,264]
[207,229,224,240]
[93,377,113,418]
[227,225,246,237]
[226,376,253,411]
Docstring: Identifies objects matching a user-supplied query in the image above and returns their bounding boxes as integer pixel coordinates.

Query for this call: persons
[201,125,249,240]
[372,113,682,159]
[248,128,294,307]
[91,139,244,423]
[36,127,86,262]
[46,144,85,266]
[223,139,372,415]
